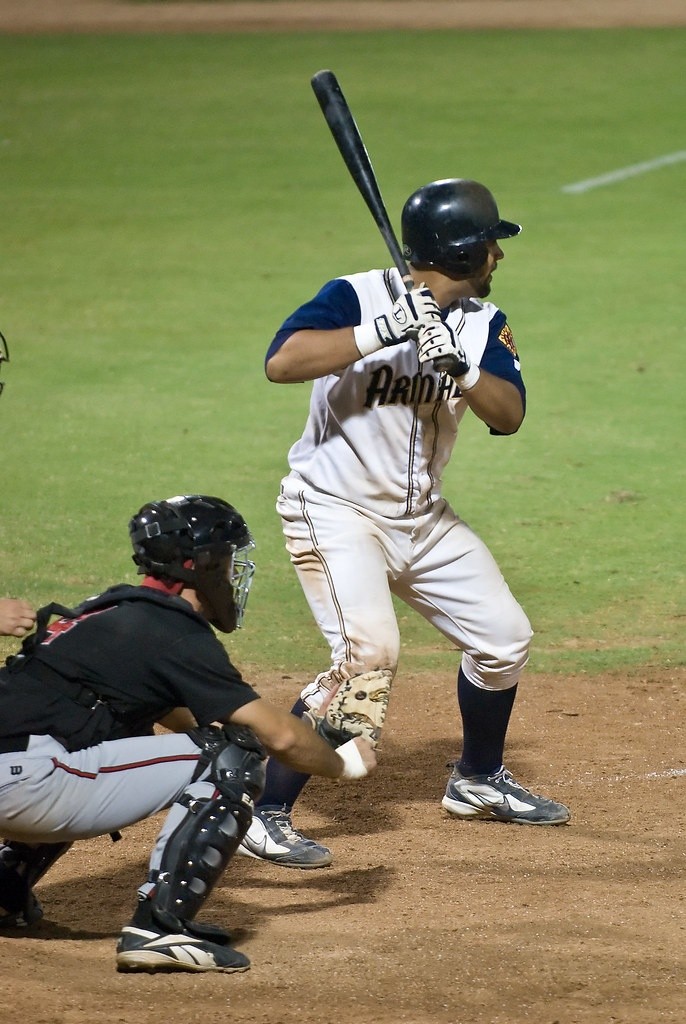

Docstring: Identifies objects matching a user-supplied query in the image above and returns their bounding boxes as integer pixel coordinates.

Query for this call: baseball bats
[310,70,455,373]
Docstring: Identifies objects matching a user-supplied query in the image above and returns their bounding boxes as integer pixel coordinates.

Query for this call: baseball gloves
[320,670,393,753]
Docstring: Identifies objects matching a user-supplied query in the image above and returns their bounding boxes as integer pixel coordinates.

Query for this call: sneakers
[116,926,249,973]
[440,766,570,825]
[235,805,332,869]
[0,852,45,930]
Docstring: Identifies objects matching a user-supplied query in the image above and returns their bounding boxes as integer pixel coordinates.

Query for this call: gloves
[414,315,470,379]
[372,288,443,346]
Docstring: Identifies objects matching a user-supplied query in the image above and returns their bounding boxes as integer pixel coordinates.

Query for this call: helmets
[130,494,254,582]
[401,178,522,275]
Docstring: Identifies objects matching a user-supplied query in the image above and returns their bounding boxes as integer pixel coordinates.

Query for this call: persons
[0,337,379,972]
[234,178,571,868]
